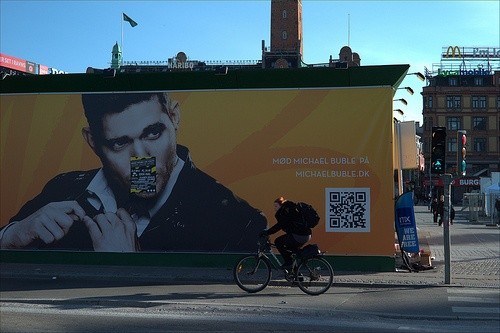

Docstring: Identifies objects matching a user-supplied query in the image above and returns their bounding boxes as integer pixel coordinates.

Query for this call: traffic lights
[458,130,468,176]
[432,126,447,175]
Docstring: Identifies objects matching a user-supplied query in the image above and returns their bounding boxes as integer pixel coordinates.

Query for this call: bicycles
[233,233,335,296]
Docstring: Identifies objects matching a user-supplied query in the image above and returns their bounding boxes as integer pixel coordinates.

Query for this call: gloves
[259,228,268,237]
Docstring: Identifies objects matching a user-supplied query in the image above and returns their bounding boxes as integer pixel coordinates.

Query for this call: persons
[259,197,312,282]
[0,94,271,253]
[432,195,455,226]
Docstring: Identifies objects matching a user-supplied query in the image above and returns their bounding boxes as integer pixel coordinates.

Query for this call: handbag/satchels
[302,243,322,256]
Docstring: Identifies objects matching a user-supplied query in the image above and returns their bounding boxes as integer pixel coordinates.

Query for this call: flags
[123,13,138,27]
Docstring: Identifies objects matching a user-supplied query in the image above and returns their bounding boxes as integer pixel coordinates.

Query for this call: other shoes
[279,253,295,269]
[292,273,304,285]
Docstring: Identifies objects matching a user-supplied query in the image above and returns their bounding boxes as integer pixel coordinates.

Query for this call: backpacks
[295,201,321,229]
